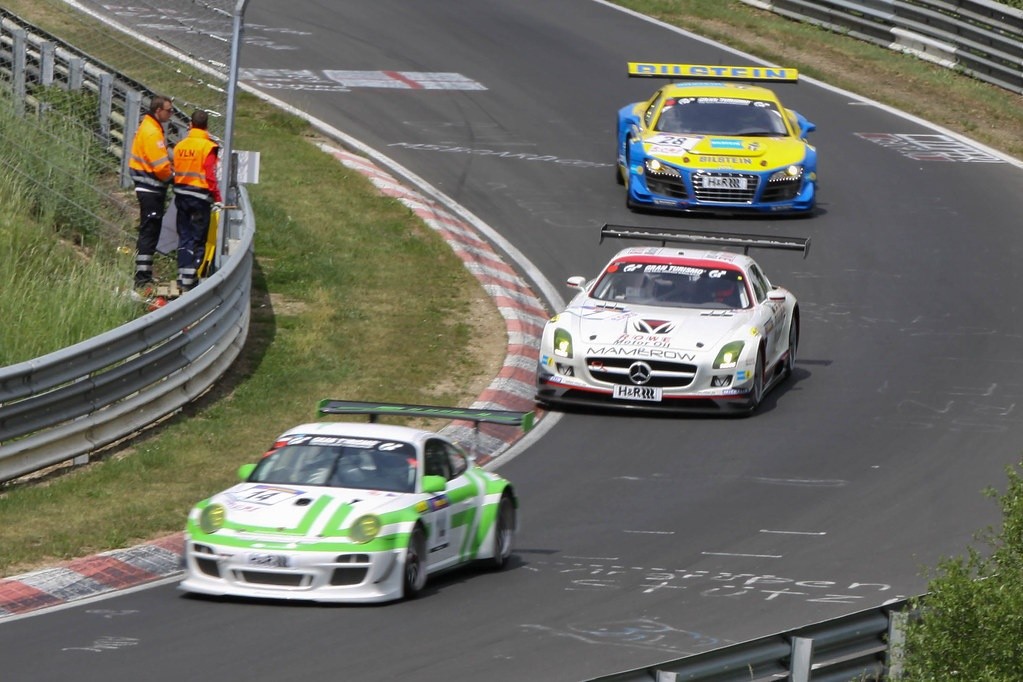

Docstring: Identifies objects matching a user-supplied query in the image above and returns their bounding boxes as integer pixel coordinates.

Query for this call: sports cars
[615,60,820,218]
[532,222,812,417]
[178,396,537,607]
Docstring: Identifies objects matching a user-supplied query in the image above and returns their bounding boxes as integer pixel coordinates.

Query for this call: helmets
[736,106,762,126]
[380,454,417,488]
[650,275,685,302]
[702,273,736,301]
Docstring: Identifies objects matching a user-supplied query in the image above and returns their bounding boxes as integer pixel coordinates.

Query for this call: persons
[172,110,222,291]
[128,96,174,298]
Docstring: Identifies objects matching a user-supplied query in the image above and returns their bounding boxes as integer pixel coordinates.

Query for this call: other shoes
[177,280,198,294]
[134,280,155,293]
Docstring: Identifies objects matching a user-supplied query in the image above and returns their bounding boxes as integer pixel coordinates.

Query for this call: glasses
[162,108,174,113]
